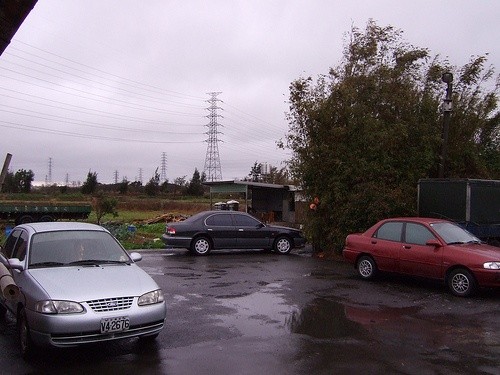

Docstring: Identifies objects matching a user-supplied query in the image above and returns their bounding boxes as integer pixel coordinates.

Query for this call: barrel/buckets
[227,200,239,211]
[215,202,227,212]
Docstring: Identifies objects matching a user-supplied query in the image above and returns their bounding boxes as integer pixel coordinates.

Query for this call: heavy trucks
[0,204,93,225]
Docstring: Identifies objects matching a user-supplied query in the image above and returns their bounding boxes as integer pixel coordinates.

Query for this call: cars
[342,217,500,298]
[161,209,308,257]
[0,221,167,363]
